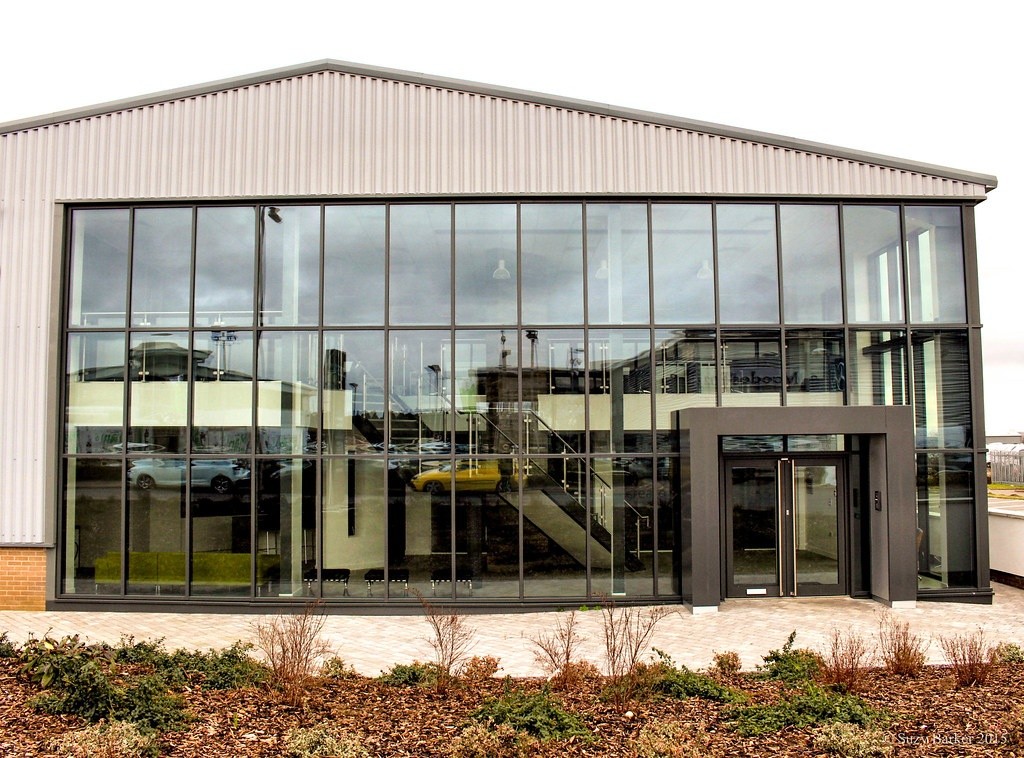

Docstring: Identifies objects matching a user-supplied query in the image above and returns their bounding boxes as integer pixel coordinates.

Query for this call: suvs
[930,451,975,484]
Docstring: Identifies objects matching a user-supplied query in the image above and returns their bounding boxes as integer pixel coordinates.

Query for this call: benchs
[93,552,272,597]
[364,569,409,596]
[430,569,473,597]
[303,569,351,597]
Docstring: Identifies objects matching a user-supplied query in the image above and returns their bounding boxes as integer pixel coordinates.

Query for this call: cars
[115,437,671,493]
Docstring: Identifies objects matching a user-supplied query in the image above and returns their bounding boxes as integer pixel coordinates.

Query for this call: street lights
[350,382,358,417]
[527,330,536,409]
[427,364,441,416]
